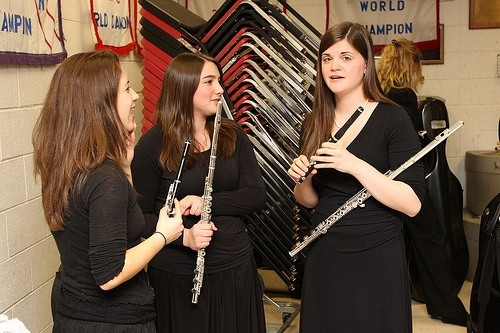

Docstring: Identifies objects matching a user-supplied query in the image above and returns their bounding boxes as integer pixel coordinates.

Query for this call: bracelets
[155,231,167,245]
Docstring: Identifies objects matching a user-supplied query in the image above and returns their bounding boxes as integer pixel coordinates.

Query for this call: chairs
[139,0,323,296]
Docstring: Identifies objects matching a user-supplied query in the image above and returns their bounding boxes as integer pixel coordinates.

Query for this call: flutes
[296,105,364,185]
[165,141,191,215]
[191,101,224,304]
[289,120,465,258]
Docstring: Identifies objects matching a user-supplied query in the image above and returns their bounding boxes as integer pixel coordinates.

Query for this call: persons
[33,51,184,333]
[378,39,423,145]
[134,53,268,333]
[289,22,427,333]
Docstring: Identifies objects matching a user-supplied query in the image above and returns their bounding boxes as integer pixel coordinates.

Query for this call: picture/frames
[417,23,445,66]
